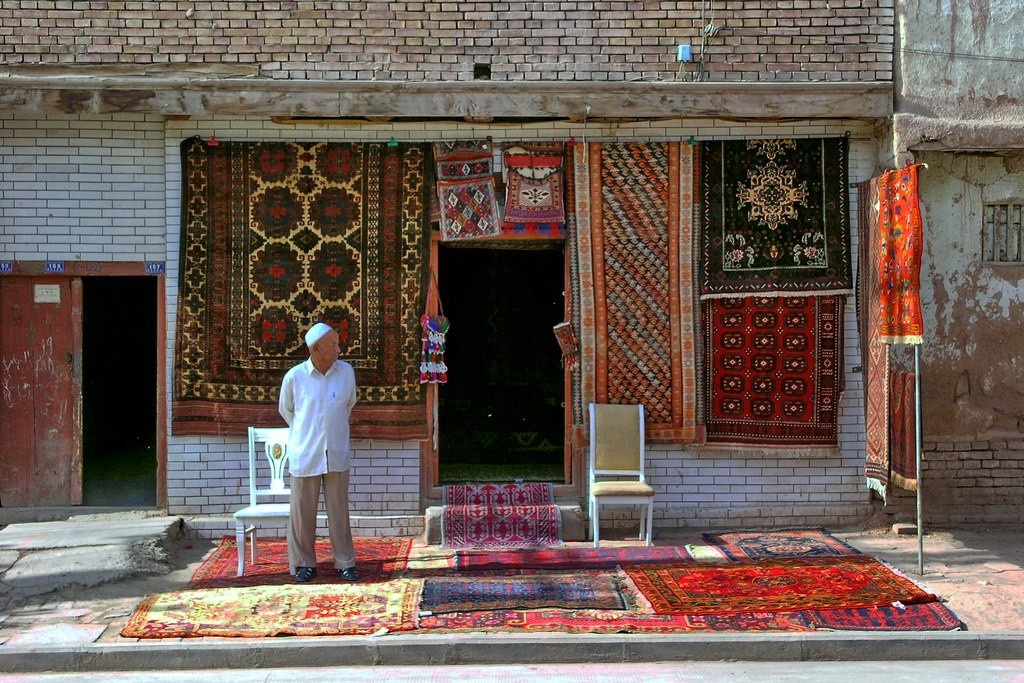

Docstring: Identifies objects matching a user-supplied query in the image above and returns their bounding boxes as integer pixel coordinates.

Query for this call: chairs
[590,403,655,547]
[234,426,296,577]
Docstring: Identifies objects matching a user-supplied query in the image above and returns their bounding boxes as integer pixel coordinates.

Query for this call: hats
[304,322,333,348]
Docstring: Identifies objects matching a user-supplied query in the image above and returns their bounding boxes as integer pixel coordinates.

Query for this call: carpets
[119,483,965,638]
[171,137,923,505]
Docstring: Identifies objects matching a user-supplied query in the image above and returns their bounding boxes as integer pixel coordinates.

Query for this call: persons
[278,323,360,581]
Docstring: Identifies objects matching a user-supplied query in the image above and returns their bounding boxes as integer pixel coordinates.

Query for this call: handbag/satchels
[420,314,450,384]
[553,321,580,372]
[432,141,567,243]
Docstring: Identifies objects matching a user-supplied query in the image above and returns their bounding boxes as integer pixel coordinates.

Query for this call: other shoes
[336,566,359,581]
[295,567,316,582]
